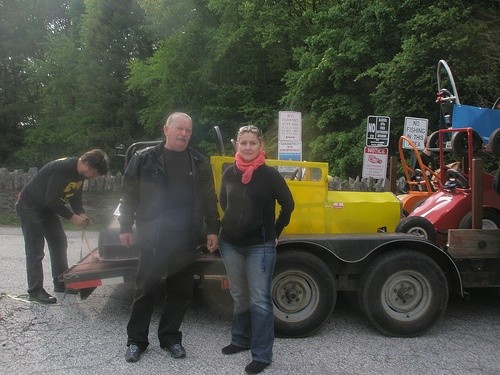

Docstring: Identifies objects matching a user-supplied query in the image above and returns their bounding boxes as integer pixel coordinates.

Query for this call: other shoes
[245,360,271,374]
[222,343,251,354]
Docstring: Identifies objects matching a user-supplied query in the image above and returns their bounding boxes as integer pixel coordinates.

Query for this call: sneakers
[125,344,146,362]
[160,340,186,358]
[29,288,57,303]
[54,283,78,294]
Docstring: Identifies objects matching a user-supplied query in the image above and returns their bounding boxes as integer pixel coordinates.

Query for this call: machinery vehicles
[58,58,500,337]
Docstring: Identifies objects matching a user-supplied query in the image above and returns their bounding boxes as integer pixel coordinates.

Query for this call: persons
[118,112,221,362]
[402,149,432,194]
[219,126,295,374]
[15,148,110,303]
